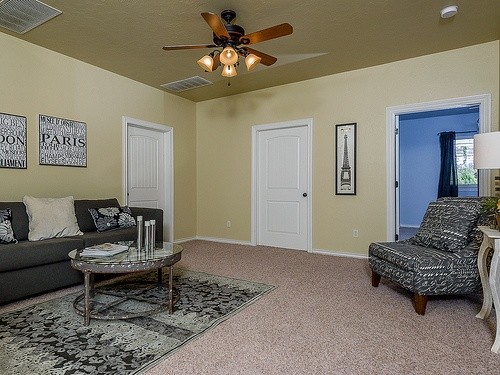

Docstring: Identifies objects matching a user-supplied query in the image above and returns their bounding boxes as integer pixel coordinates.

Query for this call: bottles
[137,216,144,249]
[144,221,150,249]
[150,220,156,248]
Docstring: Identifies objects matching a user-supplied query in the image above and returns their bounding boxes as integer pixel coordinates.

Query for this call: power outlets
[226,220,231,227]
[354,229,360,237]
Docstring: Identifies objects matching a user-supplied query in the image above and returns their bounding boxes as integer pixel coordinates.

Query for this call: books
[80,243,128,255]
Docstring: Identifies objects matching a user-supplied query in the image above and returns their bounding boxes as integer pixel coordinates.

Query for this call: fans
[164,9,293,66]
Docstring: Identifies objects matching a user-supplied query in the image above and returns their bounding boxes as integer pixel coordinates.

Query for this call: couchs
[0,198,164,308]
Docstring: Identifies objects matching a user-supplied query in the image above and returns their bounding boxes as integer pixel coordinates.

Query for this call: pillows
[0,208,18,244]
[89,205,135,232]
[23,195,84,241]
[418,200,480,252]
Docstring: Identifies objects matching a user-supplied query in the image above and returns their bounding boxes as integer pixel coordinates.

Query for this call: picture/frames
[39,114,88,167]
[334,120,359,196]
[0,112,27,169]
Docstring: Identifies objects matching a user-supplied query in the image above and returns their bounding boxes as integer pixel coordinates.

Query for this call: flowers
[483,197,496,214]
[495,198,500,212]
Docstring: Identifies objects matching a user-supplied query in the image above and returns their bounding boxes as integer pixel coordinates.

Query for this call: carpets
[0,268,275,375]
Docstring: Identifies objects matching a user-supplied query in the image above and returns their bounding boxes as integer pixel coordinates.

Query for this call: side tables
[477,225,500,355]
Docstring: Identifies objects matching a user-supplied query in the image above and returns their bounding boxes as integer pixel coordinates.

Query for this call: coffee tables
[68,242,185,327]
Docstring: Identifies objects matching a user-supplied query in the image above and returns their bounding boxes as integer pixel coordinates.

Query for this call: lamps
[196,44,261,77]
[474,130,500,170]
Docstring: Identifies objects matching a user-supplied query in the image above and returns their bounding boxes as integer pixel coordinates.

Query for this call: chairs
[368,195,497,317]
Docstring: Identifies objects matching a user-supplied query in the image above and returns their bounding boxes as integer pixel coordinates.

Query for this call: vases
[487,211,496,230]
[495,211,500,231]
